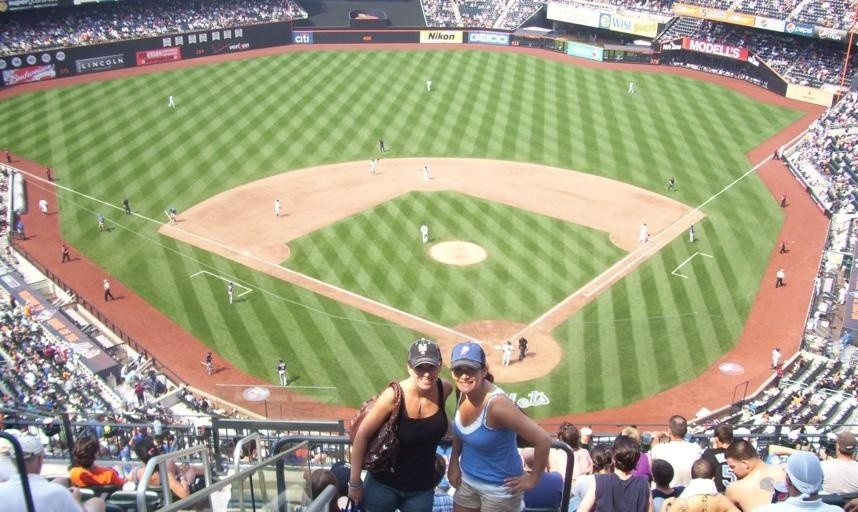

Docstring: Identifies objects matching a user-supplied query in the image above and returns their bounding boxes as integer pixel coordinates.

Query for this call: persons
[421,224,428,243]
[169,96,176,108]
[668,175,675,192]
[639,223,650,243]
[687,92,858,461]
[0,337,857,511]
[1,148,272,464]
[276,199,282,216]
[0,0,308,57]
[421,0,858,92]
[369,139,433,181]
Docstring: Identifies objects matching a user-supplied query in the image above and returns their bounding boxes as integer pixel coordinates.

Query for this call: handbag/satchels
[350,381,402,474]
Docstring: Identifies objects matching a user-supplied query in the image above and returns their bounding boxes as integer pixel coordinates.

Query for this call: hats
[786,450,824,495]
[0,429,44,459]
[449,342,485,370]
[408,338,442,368]
